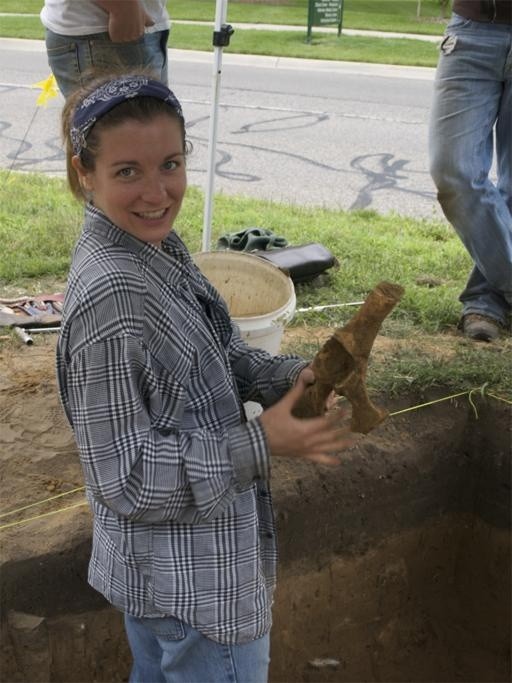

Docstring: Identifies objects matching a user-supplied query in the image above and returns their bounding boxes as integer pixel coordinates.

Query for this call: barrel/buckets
[189,250,296,420]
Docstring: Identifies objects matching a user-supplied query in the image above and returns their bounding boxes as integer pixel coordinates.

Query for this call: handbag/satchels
[255,242,336,285]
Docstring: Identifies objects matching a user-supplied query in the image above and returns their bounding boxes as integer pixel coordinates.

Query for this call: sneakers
[464,313,502,341]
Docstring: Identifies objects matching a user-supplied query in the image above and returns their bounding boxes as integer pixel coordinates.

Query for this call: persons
[40,0,171,100]
[428,1,511,340]
[56,70,355,682]
